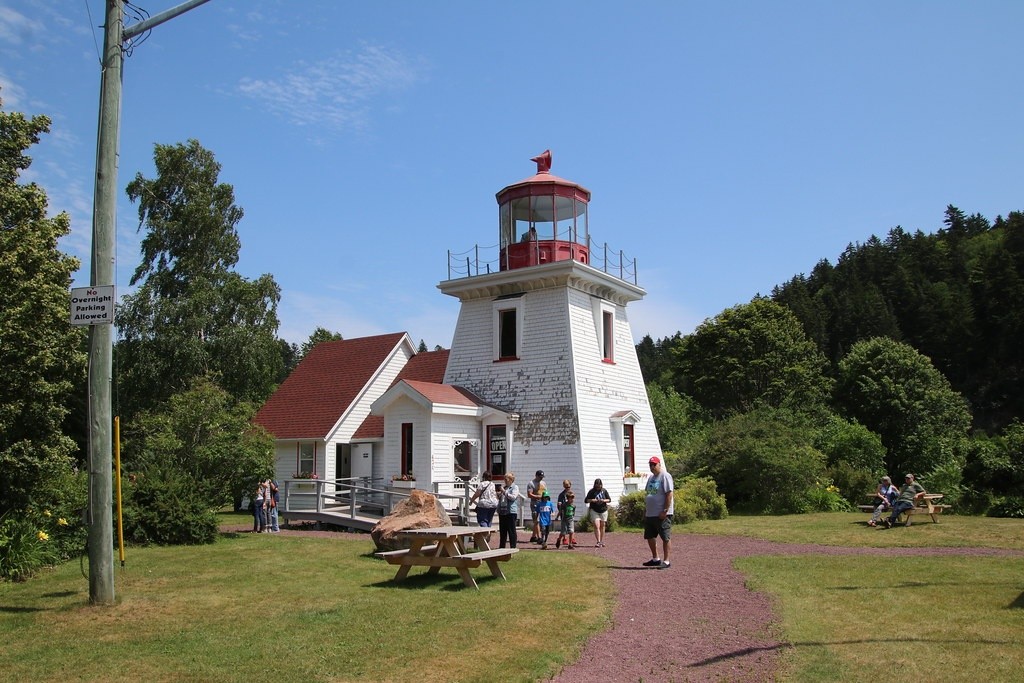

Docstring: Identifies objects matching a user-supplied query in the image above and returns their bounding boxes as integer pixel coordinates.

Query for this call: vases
[392,480,416,489]
[295,484,315,490]
[624,478,648,484]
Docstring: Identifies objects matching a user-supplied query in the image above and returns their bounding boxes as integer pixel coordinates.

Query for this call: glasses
[906,477,910,479]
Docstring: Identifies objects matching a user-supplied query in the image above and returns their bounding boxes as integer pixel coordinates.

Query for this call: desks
[387,526,506,590]
[866,493,944,527]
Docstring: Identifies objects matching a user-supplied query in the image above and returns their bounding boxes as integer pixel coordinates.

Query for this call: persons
[496,472,520,549]
[526,470,548,544]
[880,474,926,529]
[867,476,899,527]
[252,477,281,535]
[533,491,555,549]
[584,479,612,548]
[643,457,674,568]
[454,447,470,472]
[469,470,499,527]
[554,494,576,549]
[556,479,578,545]
[521,226,536,241]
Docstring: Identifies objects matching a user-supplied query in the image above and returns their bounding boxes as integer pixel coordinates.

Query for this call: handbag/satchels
[873,496,884,506]
[271,499,275,508]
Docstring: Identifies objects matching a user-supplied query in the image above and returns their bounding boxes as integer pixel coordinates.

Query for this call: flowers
[291,471,320,480]
[390,474,416,483]
[624,471,648,478]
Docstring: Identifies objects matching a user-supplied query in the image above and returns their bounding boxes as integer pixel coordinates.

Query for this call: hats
[536,470,545,477]
[905,474,914,478]
[542,491,550,497]
[648,457,660,464]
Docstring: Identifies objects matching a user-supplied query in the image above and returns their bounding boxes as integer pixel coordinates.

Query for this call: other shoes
[643,559,661,567]
[568,545,576,550]
[868,521,876,527]
[656,561,670,570]
[563,538,568,544]
[571,538,577,544]
[530,536,538,542]
[537,538,543,544]
[542,542,547,549]
[596,541,605,547]
[879,517,892,529]
[251,527,280,534]
[556,538,561,549]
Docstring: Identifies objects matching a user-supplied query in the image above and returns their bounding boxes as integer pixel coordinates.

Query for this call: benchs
[450,548,520,568]
[857,505,952,515]
[373,545,438,564]
